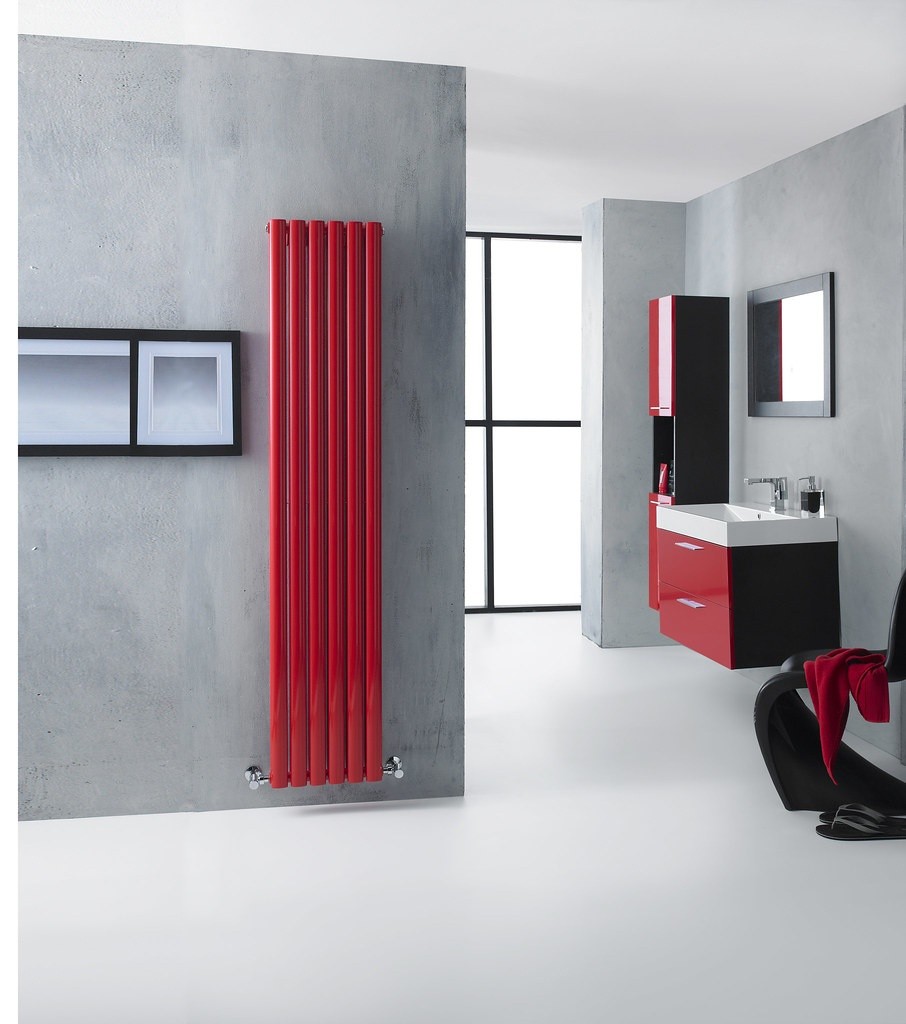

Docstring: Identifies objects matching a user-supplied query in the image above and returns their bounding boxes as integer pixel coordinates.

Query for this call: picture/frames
[18,324,246,460]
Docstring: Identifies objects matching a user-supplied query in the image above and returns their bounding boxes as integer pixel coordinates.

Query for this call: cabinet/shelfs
[646,294,733,612]
[651,503,847,671]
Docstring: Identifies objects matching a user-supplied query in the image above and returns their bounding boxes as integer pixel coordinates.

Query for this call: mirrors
[745,270,839,423]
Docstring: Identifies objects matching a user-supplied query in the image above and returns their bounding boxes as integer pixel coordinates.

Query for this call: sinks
[656,502,839,547]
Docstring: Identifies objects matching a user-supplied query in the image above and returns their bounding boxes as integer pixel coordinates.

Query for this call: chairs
[751,568,906,814]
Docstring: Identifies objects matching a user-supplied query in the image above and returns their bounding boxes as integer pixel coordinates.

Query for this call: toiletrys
[799,476,825,519]
[658,460,674,495]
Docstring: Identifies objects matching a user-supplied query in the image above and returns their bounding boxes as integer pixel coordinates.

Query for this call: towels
[802,642,890,785]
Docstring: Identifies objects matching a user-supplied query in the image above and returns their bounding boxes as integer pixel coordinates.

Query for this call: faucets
[744,477,788,511]
